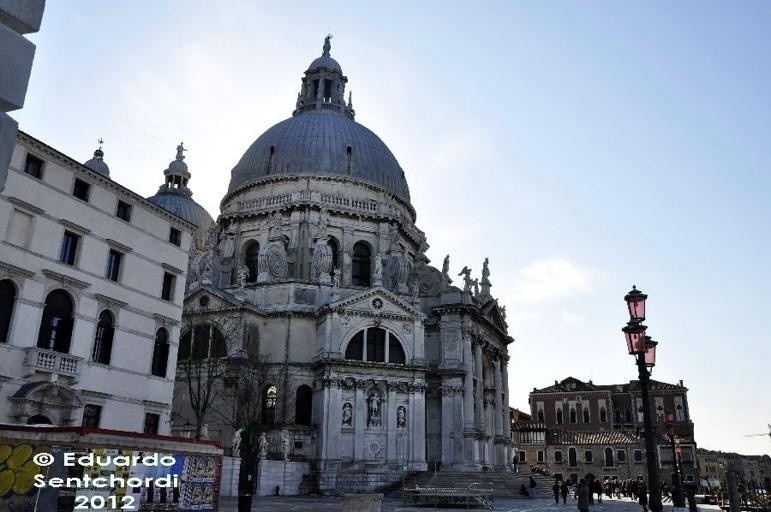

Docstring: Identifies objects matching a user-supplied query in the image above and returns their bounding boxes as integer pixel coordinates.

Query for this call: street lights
[664,409,683,488]
[622,285,663,512]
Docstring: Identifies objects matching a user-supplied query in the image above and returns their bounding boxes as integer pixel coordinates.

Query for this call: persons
[510,454,673,512]
